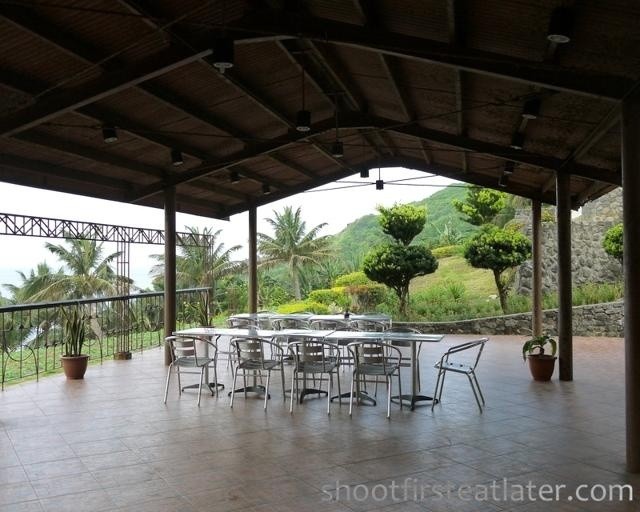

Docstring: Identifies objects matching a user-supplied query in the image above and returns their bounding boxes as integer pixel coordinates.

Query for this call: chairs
[162,310,422,425]
[429,336,489,414]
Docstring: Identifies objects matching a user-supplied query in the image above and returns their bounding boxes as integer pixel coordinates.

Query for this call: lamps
[510,131,524,150]
[171,151,185,168]
[101,124,119,144]
[211,37,235,74]
[293,70,384,193]
[520,98,540,121]
[546,1,571,44]
[497,161,517,188]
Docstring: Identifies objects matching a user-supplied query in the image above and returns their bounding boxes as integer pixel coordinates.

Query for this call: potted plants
[59,309,91,379]
[522,334,558,382]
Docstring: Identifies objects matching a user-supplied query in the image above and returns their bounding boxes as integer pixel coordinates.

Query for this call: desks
[170,326,444,411]
